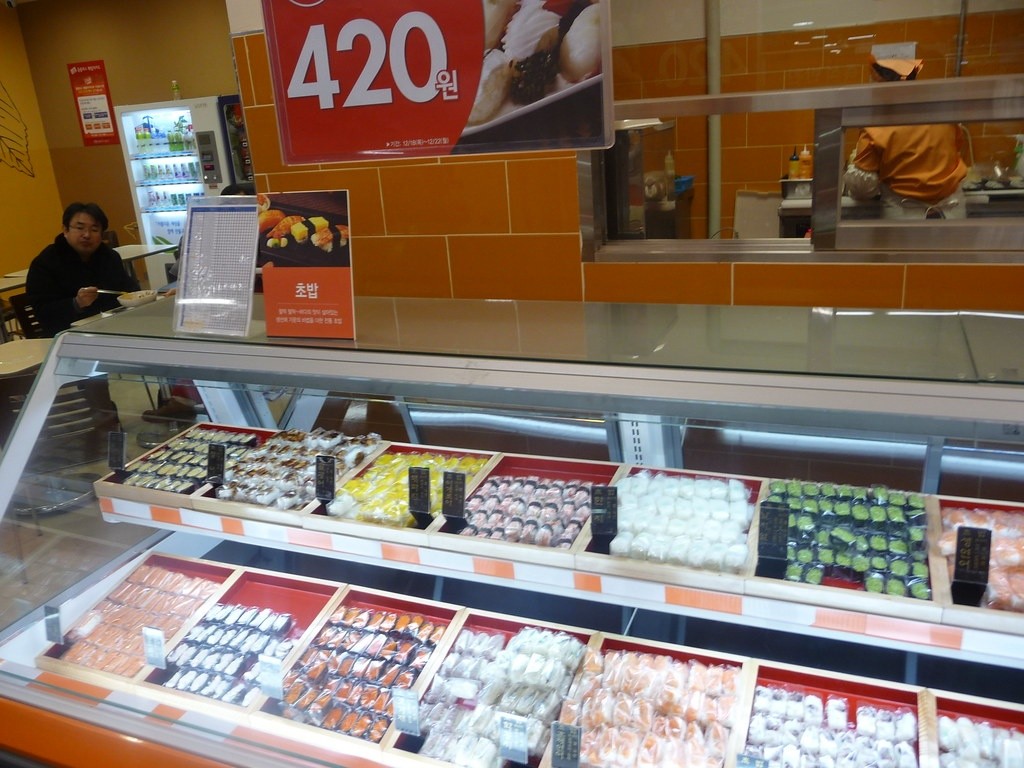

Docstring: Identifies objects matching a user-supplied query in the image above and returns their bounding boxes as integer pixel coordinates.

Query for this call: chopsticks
[81,288,127,295]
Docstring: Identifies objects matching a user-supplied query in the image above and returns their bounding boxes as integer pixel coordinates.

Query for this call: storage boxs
[674,176,694,192]
[778,174,814,200]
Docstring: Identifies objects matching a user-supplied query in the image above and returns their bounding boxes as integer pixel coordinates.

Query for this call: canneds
[170,192,204,206]
[142,161,201,180]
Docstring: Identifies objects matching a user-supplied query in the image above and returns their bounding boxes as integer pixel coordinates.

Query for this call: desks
[0,243,177,378]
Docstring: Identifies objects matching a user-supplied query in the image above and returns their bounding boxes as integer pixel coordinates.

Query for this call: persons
[24,201,143,336]
[140,184,255,423]
[841,54,967,222]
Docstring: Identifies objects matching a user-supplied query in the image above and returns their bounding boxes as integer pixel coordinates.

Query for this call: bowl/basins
[117,290,157,307]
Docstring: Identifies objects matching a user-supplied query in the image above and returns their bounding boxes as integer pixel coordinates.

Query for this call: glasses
[68,223,104,238]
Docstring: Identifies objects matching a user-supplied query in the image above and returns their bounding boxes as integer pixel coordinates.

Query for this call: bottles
[800,144,813,179]
[134,127,203,208]
[665,150,675,194]
[787,146,799,179]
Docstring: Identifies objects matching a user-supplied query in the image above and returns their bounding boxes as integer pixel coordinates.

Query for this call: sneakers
[142,397,196,422]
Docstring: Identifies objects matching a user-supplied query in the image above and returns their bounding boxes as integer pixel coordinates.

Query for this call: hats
[867,58,923,85]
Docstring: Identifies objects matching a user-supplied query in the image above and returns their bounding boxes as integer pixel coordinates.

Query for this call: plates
[460,71,604,137]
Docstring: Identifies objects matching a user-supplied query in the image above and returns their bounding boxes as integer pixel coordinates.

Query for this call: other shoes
[94,417,123,453]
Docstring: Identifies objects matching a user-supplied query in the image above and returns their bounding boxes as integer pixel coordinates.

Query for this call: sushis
[256,195,348,254]
[463,0,603,125]
[120,427,1023,616]
[59,565,1024,768]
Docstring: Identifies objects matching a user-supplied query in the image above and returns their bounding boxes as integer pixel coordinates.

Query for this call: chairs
[9,292,52,339]
